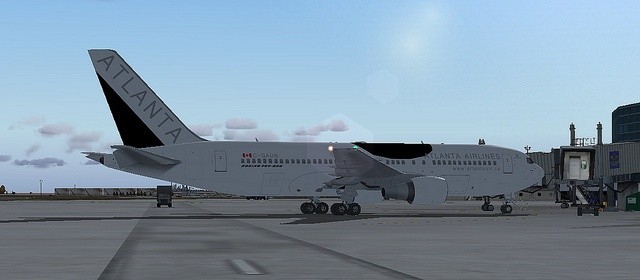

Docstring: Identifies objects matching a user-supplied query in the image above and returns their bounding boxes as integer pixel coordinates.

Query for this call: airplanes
[81,49,544,215]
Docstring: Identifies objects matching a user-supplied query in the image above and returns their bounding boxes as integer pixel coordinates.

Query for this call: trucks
[157,186,174,207]
